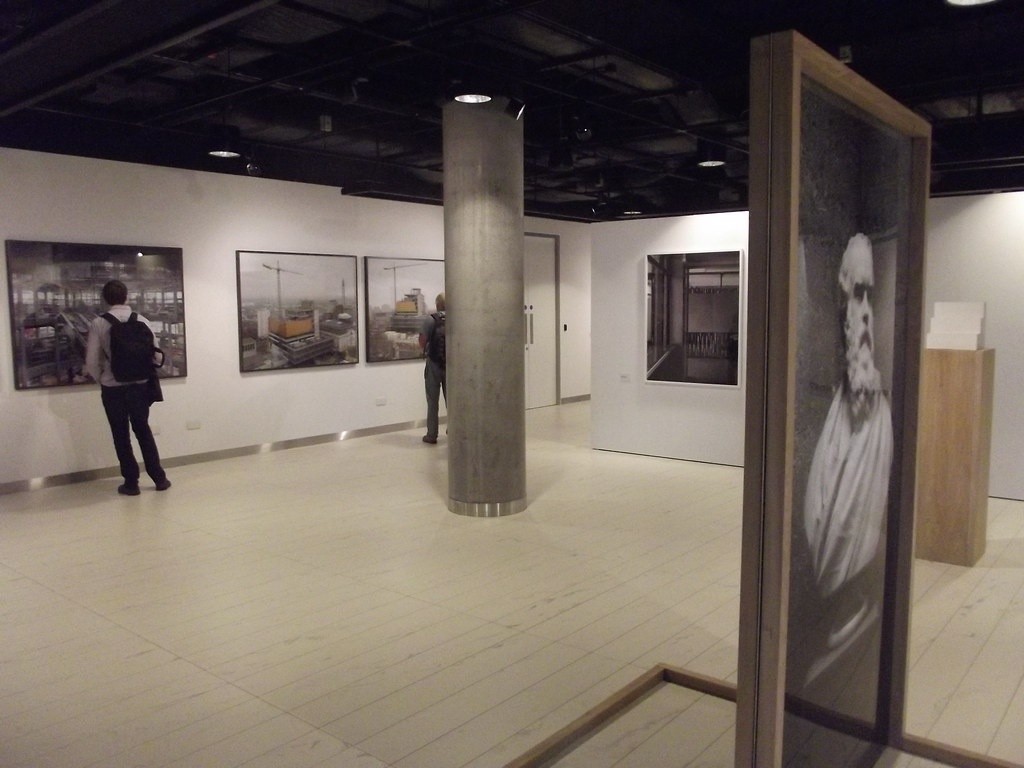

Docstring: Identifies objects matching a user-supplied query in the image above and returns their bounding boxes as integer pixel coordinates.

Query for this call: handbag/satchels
[150,346,165,402]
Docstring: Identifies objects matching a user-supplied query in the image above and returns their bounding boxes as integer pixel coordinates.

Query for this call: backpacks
[99,310,154,382]
[428,313,446,365]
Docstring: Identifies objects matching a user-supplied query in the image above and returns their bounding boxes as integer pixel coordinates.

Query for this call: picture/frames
[235,250,359,372]
[364,256,445,363]
[4,239,187,390]
[733,28,932,768]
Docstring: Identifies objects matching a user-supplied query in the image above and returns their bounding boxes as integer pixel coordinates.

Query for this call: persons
[418,293,448,444]
[86,280,171,495]
[801,235,896,594]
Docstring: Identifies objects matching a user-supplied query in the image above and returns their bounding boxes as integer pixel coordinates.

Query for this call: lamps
[207,124,242,158]
[454,72,492,104]
[504,97,525,121]
[697,126,727,166]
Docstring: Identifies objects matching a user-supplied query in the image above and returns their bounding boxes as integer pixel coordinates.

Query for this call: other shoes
[156,479,170,491]
[423,436,437,445]
[118,484,141,496]
